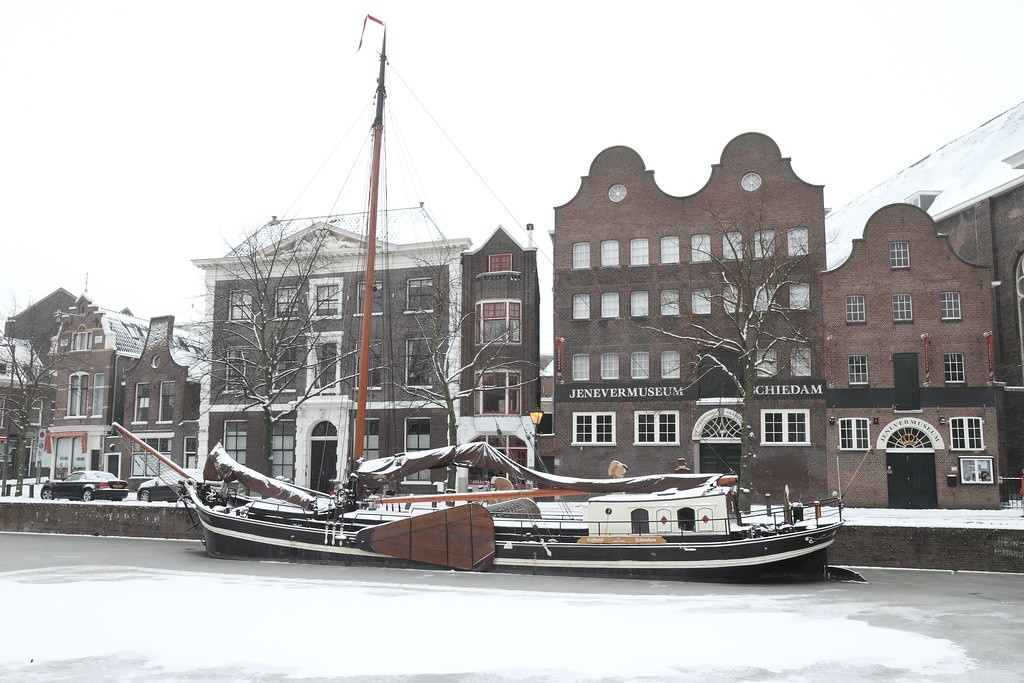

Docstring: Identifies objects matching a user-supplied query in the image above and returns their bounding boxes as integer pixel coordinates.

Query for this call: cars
[137,469,203,504]
[41,470,129,502]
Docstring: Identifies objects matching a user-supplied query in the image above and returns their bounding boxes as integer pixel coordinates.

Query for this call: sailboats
[108,11,869,587]
